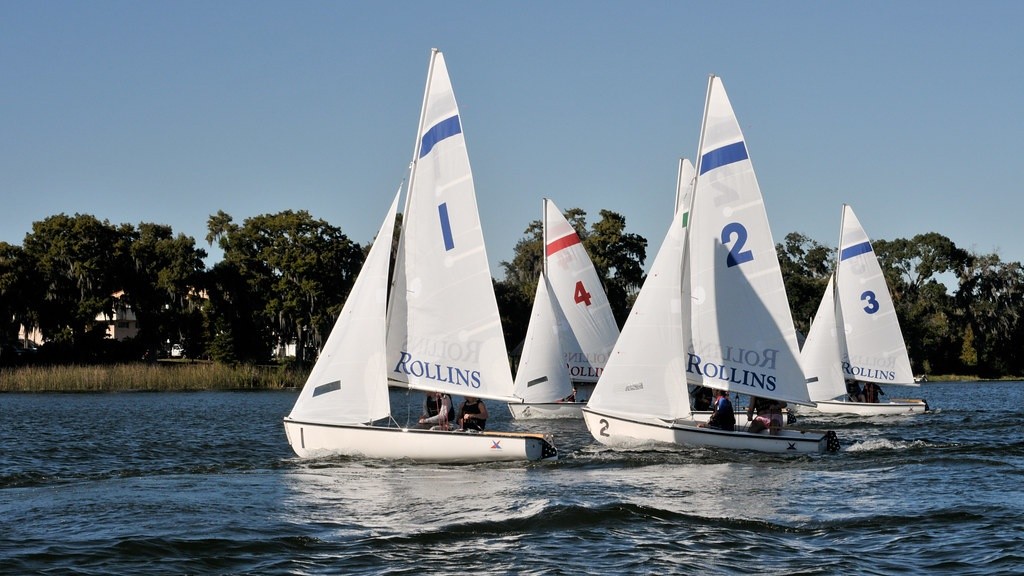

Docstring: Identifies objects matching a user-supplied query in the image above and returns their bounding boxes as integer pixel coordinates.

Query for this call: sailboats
[801,200,931,417]
[287,53,556,462]
[504,196,621,419]
[580,76,843,456]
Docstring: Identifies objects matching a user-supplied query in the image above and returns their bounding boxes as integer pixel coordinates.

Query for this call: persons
[411,392,454,430]
[861,381,885,402]
[689,385,736,432]
[746,395,787,436]
[456,397,487,433]
[845,379,862,402]
